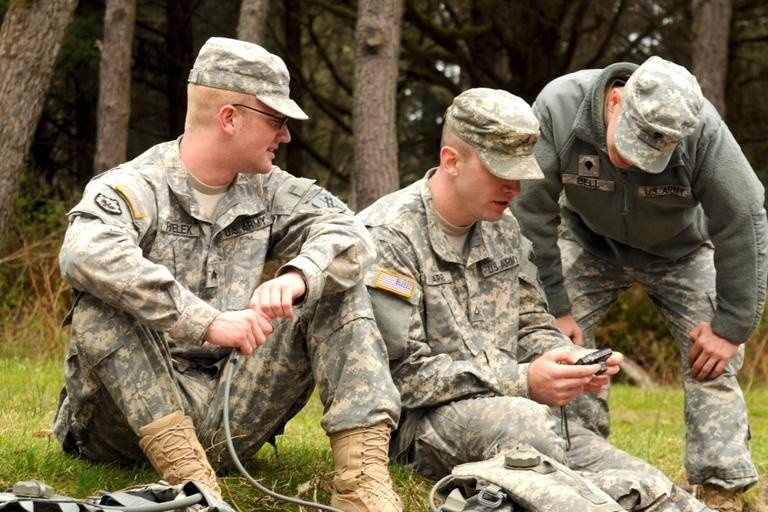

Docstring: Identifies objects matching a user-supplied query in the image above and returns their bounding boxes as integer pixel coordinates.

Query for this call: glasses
[231,104,289,128]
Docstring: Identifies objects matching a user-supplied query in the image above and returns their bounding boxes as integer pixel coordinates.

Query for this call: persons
[50,34,406,512]
[504,54,768,512]
[354,84,719,512]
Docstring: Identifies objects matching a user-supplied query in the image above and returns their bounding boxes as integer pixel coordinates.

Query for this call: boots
[329,423,404,512]
[138,411,222,512]
[696,486,743,511]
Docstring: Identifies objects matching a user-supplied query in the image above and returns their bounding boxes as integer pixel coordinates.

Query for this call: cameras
[575,348,613,376]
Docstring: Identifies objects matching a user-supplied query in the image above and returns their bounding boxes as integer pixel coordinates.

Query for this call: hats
[187,37,309,121]
[614,56,704,174]
[443,88,545,181]
